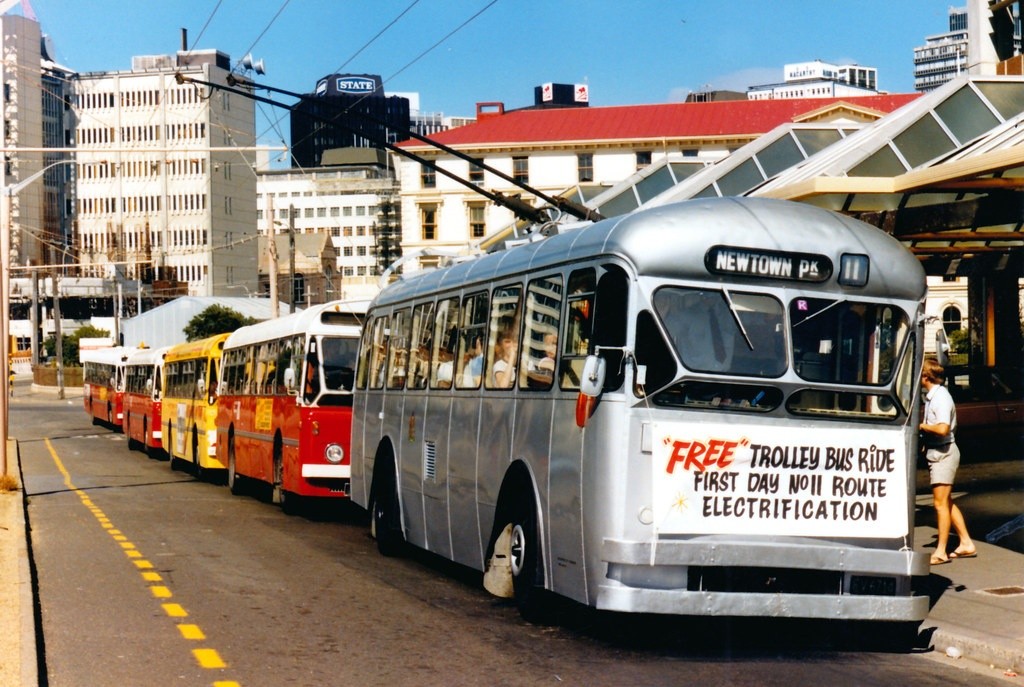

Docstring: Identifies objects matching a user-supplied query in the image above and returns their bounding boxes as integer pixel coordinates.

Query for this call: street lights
[1,159,107,436]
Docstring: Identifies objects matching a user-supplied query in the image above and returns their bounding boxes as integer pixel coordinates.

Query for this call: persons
[436,330,518,389]
[534,328,558,372]
[919,358,977,565]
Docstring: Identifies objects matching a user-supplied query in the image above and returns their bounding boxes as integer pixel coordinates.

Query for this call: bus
[83,296,369,505]
[350,197,930,643]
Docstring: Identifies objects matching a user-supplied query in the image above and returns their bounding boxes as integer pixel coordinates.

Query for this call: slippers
[947,551,978,558]
[930,556,953,565]
